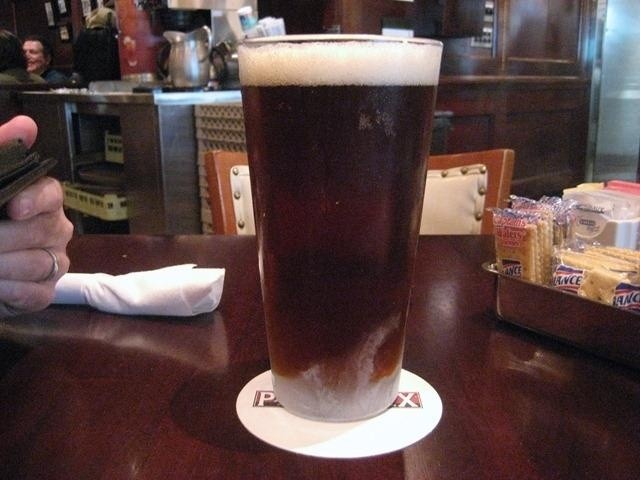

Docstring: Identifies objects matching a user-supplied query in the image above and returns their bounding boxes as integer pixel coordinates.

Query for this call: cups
[235,34,443,426]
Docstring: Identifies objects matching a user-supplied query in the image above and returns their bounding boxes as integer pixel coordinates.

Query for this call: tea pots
[164,27,215,91]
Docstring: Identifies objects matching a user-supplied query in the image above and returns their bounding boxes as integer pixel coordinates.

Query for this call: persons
[74,0,118,88]
[21,35,67,84]
[0,28,47,125]
[0,115,75,321]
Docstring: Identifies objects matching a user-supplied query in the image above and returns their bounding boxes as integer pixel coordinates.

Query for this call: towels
[46,261,230,320]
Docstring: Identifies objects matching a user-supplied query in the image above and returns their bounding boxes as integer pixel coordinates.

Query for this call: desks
[0,227,640,480]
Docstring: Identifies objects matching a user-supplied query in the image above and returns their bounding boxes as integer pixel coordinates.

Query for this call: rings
[44,248,58,281]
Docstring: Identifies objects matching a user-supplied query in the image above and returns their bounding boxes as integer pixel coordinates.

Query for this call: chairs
[205,142,518,240]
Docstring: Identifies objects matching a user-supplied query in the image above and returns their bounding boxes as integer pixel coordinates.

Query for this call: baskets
[103,128,124,165]
[58,181,127,223]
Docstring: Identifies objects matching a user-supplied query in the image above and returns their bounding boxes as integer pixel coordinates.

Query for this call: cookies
[493,203,639,306]
